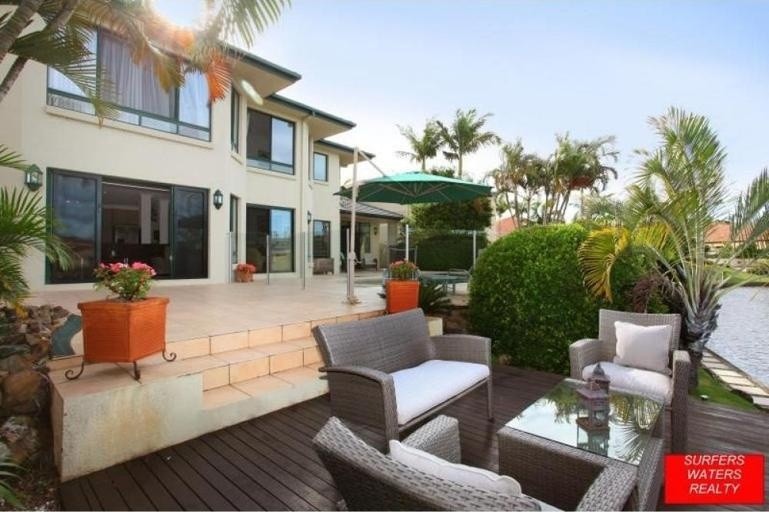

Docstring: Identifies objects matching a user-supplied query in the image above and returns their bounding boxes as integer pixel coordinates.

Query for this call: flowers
[388,260,419,281]
[92,262,157,302]
[237,264,256,273]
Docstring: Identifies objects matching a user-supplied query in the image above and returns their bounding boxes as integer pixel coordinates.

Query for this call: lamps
[308,210,312,224]
[374,227,378,235]
[25,164,43,192]
[214,189,223,210]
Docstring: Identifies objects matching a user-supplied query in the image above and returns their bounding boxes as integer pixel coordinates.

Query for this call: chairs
[568,308,692,455]
[312,415,636,512]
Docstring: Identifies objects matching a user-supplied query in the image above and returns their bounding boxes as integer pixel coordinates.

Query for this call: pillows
[388,439,522,497]
[613,320,672,376]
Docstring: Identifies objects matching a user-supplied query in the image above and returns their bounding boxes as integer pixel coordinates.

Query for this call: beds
[497,378,670,511]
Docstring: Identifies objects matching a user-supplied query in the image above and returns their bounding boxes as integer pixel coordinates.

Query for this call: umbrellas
[333,145,495,304]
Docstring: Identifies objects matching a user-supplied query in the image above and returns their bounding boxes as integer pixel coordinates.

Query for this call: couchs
[311,307,494,453]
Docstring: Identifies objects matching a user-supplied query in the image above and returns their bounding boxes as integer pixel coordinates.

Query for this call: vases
[65,296,177,380]
[235,271,253,283]
[385,279,420,314]
